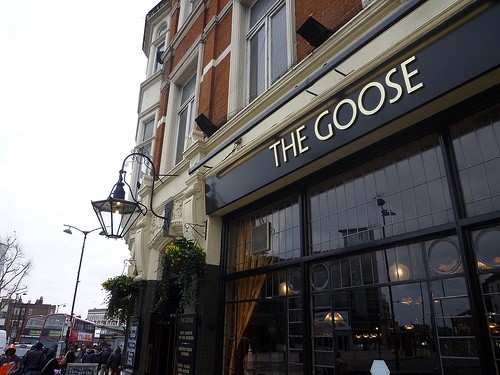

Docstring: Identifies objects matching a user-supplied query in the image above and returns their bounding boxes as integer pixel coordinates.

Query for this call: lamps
[89,151,174,238]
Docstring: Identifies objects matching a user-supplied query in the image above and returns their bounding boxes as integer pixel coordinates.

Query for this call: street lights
[61,222,113,355]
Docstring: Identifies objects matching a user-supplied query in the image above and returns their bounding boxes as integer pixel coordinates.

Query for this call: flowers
[155,238,209,326]
[98,274,145,327]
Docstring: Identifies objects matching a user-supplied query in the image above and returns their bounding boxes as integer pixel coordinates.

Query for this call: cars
[11,343,34,360]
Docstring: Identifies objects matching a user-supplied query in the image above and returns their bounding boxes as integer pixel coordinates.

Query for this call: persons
[0,341,123,375]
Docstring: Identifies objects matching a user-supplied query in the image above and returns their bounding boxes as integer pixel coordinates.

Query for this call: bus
[19,313,52,356]
[92,323,127,349]
[39,312,96,360]
